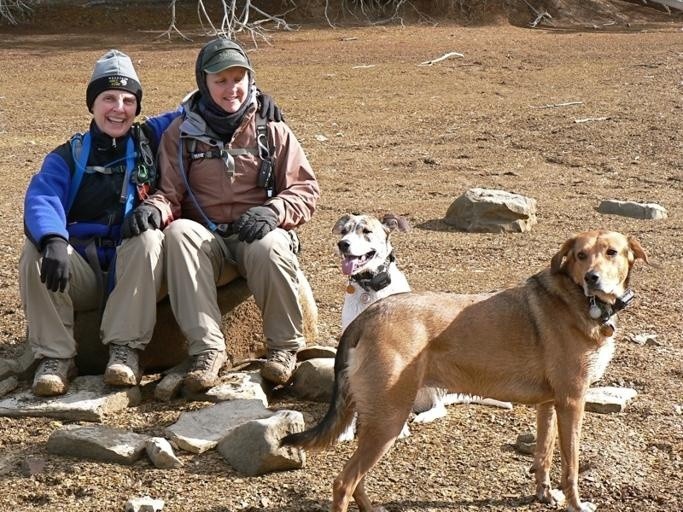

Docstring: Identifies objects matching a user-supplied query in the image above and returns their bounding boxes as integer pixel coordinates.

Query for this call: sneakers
[259,345,298,384]
[185,345,229,390]
[103,342,147,387]
[32,353,78,396]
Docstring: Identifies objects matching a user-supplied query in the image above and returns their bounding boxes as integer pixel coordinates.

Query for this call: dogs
[276,228,650,511]
[330,211,515,445]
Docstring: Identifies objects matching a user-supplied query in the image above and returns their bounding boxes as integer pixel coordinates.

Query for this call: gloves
[121,204,162,238]
[39,236,71,294]
[257,93,286,123]
[234,205,279,243]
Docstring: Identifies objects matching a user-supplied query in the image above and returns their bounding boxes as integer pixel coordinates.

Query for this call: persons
[18,48,285,396]
[122,36,321,393]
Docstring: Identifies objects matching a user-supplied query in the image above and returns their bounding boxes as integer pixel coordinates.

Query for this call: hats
[197,37,256,81]
[86,49,143,117]
